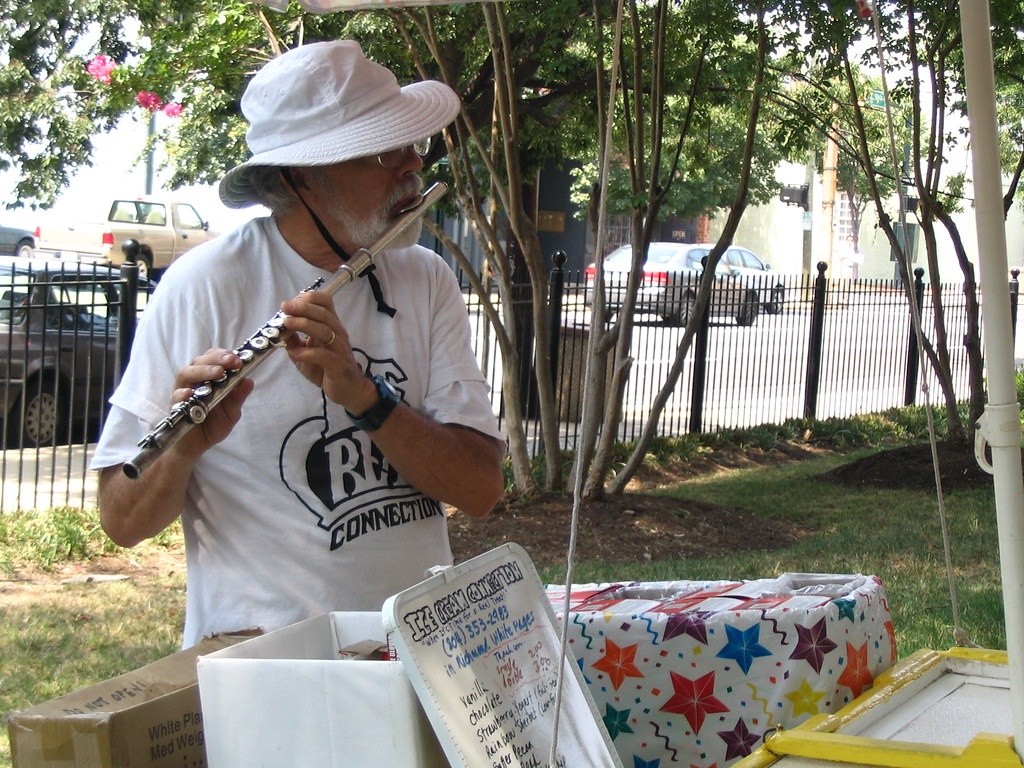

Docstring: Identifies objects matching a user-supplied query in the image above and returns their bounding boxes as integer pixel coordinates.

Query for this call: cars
[0,223,38,259]
[584,241,760,328]
[692,244,786,315]
[0,260,163,449]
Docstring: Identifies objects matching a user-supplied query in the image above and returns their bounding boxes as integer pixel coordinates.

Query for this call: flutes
[122,180,452,479]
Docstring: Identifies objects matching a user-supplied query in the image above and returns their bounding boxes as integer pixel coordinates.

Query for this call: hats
[219,39,461,209]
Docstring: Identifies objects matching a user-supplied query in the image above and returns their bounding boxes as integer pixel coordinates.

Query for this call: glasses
[378,136,431,168]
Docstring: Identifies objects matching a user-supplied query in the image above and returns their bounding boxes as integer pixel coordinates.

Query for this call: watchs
[344,375,399,431]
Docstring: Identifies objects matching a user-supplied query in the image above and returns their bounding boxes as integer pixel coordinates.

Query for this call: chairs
[2,289,90,331]
[113,208,133,223]
[146,210,165,226]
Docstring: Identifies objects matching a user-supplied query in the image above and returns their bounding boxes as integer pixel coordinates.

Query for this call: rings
[324,332,335,346]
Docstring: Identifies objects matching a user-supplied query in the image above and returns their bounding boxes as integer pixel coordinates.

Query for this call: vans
[102,195,223,272]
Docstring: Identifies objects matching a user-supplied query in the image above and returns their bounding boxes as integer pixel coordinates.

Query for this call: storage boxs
[6,542,899,768]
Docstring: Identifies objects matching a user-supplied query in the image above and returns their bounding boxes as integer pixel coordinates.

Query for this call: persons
[89,38,506,660]
